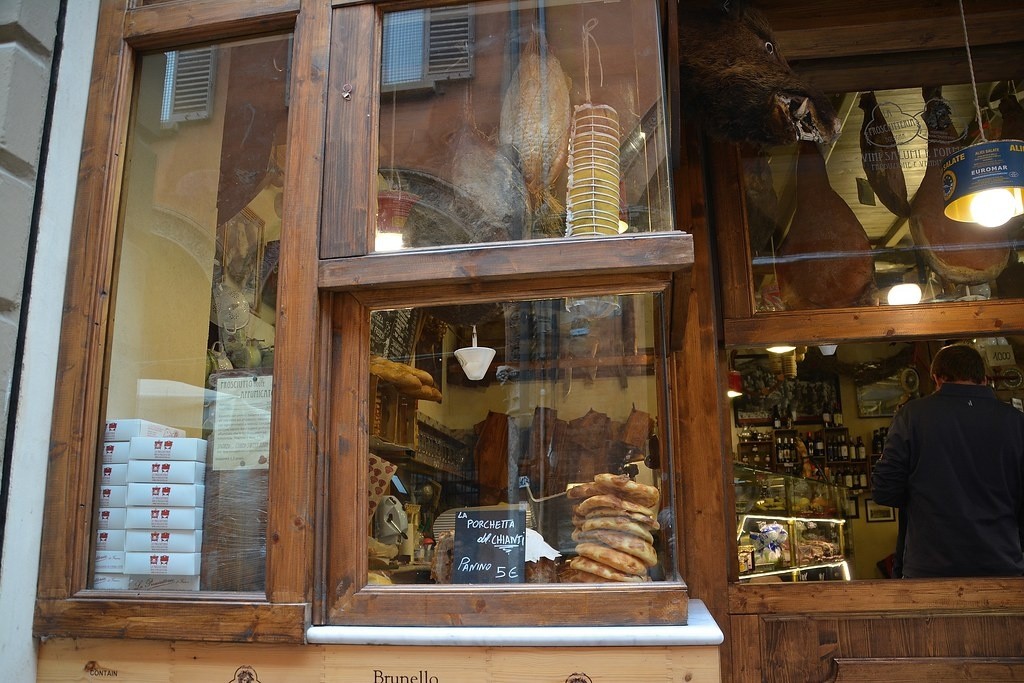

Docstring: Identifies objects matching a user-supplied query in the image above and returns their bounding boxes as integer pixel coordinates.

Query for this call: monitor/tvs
[390,475,410,503]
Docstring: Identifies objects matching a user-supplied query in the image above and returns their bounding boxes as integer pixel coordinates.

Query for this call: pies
[563,472,660,583]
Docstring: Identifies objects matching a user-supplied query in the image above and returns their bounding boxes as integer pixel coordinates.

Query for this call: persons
[870,343,1024,580]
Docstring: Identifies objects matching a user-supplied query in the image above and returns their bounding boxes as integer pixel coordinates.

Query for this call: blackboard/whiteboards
[452,510,526,583]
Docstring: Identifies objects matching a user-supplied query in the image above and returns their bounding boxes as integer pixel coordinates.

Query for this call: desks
[376,566,431,576]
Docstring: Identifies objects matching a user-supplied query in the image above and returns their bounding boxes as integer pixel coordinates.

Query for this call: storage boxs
[125,483,205,507]
[103,418,187,442]
[123,552,201,575]
[128,436,207,463]
[124,529,203,553]
[126,459,206,485]
[96,530,126,551]
[99,486,127,508]
[101,464,128,486]
[94,574,129,589]
[94,551,125,573]
[129,575,200,591]
[104,442,131,463]
[98,508,126,530]
[125,506,203,529]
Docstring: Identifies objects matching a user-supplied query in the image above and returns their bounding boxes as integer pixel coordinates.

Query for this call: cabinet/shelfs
[733,461,851,581]
[737,427,882,493]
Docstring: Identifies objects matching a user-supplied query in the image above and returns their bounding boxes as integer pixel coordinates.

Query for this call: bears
[675,0,844,146]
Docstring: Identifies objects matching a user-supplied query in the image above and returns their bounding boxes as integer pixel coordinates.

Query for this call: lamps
[454,324,497,381]
[618,180,629,233]
[941,0,1024,228]
[886,283,922,306]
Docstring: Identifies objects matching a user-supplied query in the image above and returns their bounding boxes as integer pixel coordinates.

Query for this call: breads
[368,354,443,402]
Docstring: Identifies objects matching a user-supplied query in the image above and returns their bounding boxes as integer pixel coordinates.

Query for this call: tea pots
[736,425,755,441]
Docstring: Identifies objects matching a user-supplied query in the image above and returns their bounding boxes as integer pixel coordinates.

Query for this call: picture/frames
[856,362,922,418]
[731,352,842,428]
[843,497,859,520]
[865,498,896,523]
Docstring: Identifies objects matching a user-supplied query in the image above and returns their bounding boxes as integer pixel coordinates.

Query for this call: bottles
[776,430,868,489]
[873,427,889,453]
[822,403,843,427]
[742,432,772,471]
[773,405,781,429]
[414,528,425,562]
[786,405,793,430]
